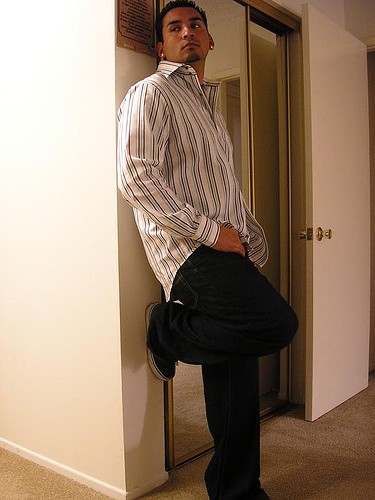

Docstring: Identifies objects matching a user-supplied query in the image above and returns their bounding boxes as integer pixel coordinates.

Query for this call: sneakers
[145,302,176,381]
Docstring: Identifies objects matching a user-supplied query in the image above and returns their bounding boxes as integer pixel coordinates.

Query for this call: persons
[114,0,302,500]
